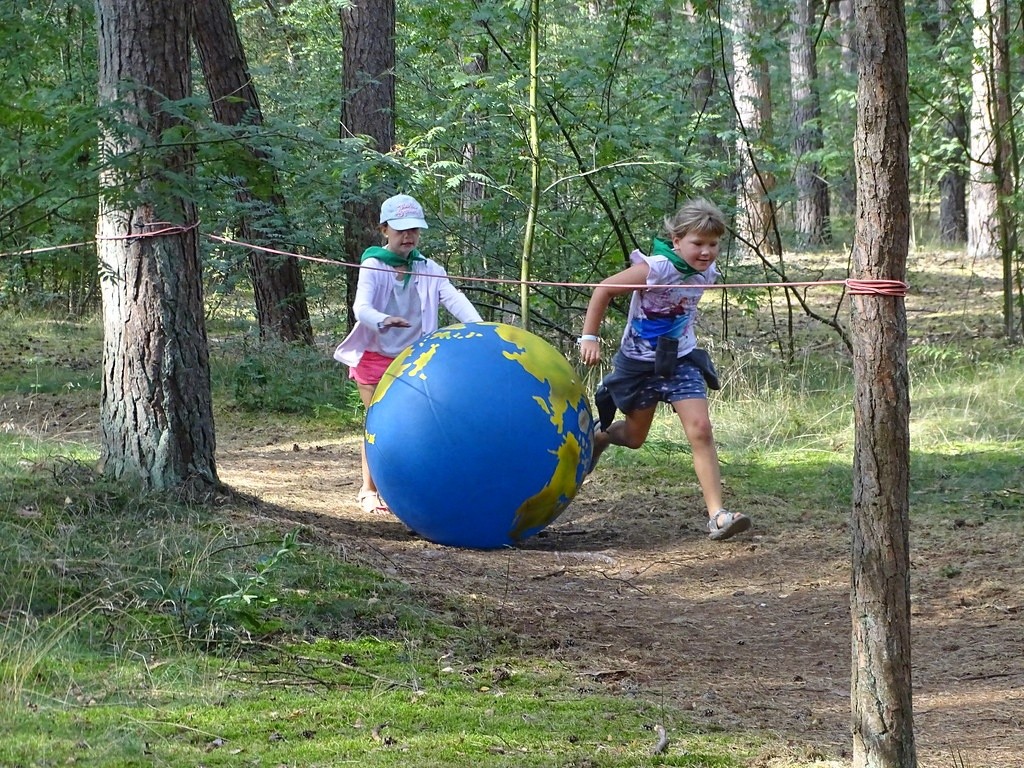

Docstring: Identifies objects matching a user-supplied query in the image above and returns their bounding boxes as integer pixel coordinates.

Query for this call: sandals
[707,508,750,541]
[587,418,600,474]
[358,488,392,516]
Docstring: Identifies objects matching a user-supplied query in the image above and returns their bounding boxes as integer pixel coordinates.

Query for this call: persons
[332,192,485,513]
[581,200,750,540]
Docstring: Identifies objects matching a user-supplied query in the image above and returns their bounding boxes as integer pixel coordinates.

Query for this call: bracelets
[576,334,602,344]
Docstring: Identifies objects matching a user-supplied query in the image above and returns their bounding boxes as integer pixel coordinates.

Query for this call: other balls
[363,321,596,550]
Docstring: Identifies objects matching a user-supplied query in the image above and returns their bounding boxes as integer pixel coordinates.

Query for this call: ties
[361,245,427,290]
[653,237,706,281]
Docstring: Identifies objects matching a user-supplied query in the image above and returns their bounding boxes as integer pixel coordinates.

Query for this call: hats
[380,194,428,231]
